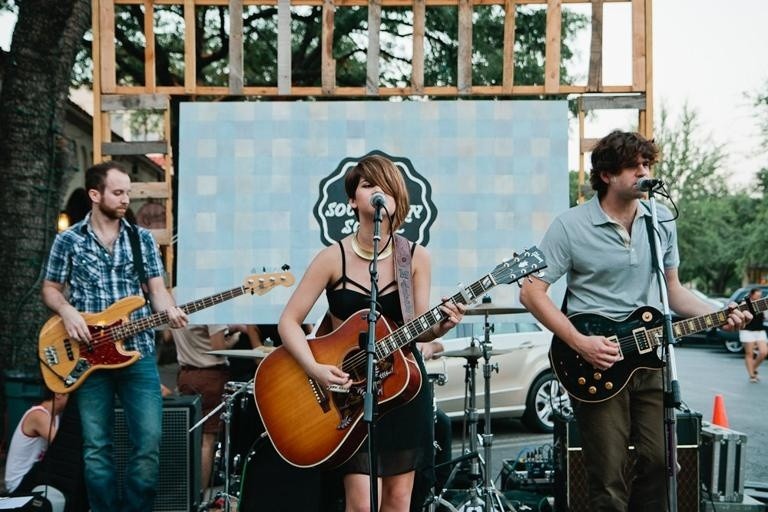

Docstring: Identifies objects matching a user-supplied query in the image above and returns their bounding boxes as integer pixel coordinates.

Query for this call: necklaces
[350,227,394,261]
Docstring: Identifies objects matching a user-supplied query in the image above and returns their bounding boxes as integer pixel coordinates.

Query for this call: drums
[211,381,267,494]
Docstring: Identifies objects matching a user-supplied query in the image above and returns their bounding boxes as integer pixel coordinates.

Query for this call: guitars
[37,264,293,394]
[549,295,768,403]
[254,245,547,470]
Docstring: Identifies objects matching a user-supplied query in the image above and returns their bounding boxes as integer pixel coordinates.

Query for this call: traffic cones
[711,396,730,429]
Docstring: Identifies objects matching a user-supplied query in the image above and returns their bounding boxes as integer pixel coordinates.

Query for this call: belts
[181,364,229,371]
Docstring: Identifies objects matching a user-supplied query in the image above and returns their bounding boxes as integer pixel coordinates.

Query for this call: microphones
[369,191,386,209]
[638,178,658,192]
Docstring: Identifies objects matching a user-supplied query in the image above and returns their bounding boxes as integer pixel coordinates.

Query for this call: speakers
[113,394,204,512]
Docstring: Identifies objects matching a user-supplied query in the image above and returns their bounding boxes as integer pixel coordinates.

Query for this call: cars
[661,284,768,360]
[415,315,576,433]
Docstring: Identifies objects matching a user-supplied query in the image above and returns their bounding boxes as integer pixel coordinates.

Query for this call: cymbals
[463,303,530,314]
[199,349,269,360]
[430,347,518,357]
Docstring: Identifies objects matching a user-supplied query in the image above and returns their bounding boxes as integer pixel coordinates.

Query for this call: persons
[2,379,75,511]
[38,160,190,510]
[275,153,469,511]
[515,130,758,511]
[736,287,768,383]
[408,339,454,495]
[152,319,318,499]
